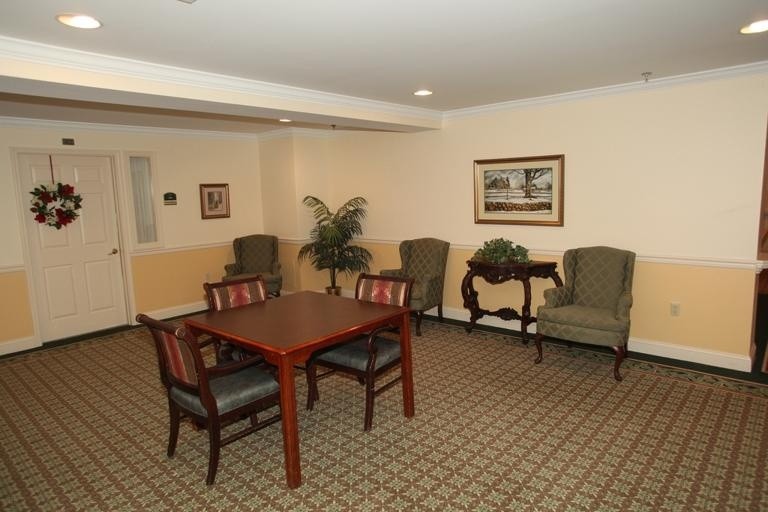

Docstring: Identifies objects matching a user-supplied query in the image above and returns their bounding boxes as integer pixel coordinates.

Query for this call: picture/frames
[472,154,565,227]
[199,182,231,219]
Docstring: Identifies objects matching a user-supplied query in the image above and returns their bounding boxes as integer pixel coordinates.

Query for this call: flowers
[28,182,83,230]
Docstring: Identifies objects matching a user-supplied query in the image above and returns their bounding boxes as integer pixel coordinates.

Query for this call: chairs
[135,313,281,485]
[204,273,284,362]
[534,245,636,381]
[222,233,282,299]
[306,273,416,431]
[380,237,451,337]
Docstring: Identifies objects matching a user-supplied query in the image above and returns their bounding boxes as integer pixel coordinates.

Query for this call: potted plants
[297,196,374,296]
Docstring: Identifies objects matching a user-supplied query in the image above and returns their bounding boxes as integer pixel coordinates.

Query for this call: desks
[183,289,417,490]
[460,259,563,345]
